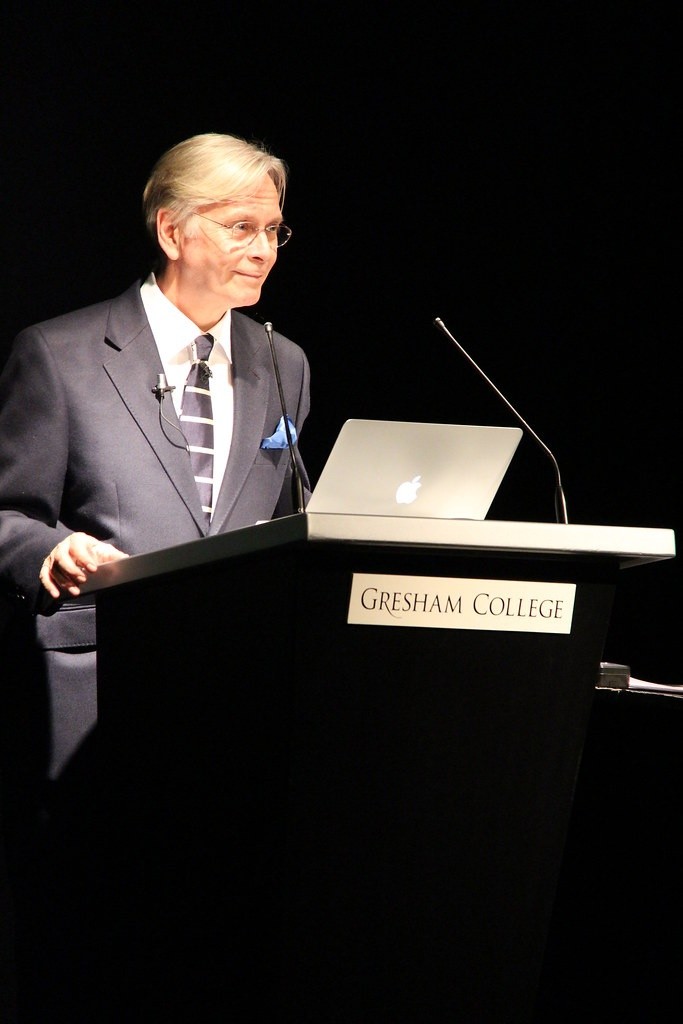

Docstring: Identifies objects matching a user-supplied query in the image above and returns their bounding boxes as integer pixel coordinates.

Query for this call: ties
[176,334,214,534]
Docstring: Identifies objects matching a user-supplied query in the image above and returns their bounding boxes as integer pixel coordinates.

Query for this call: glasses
[193,211,293,249]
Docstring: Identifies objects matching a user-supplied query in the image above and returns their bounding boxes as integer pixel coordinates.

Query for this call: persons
[0,125,319,1024]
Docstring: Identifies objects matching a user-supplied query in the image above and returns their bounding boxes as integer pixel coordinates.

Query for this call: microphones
[264,322,305,513]
[432,317,569,524]
[150,373,189,455]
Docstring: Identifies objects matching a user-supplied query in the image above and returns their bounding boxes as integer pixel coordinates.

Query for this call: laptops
[305,418,524,520]
